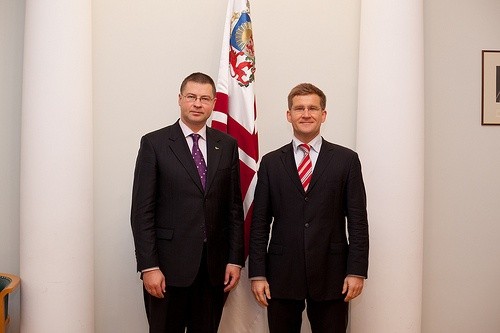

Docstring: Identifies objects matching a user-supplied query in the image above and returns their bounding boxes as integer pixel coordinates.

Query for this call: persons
[248,84,368,333]
[131,73,245,333]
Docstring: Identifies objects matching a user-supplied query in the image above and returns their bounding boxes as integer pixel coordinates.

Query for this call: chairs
[0,272,21,333]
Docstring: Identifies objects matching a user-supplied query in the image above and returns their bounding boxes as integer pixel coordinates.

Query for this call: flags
[209,0,260,305]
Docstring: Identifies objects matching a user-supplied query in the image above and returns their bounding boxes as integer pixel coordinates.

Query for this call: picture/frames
[481,49,500,126]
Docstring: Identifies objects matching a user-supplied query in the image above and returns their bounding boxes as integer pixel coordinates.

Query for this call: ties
[297,143,313,193]
[188,133,207,194]
